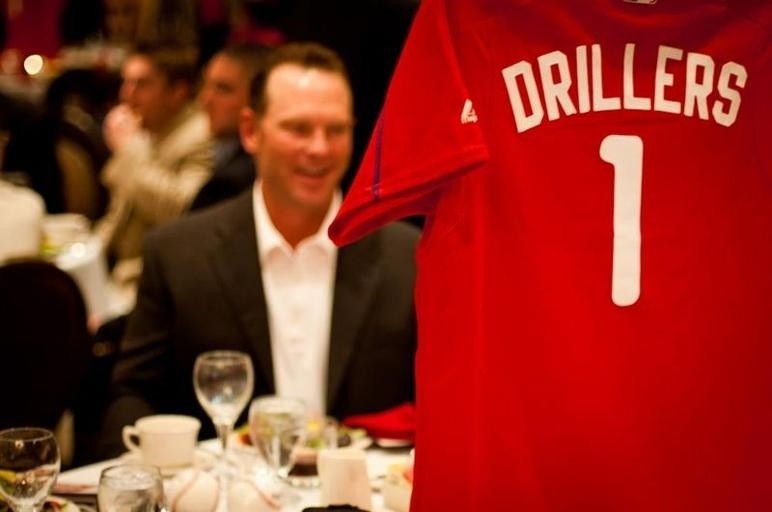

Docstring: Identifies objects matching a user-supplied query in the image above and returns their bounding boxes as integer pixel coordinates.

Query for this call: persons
[0,28,422,441]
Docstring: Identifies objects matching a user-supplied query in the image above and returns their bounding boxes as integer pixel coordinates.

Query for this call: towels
[337,399,417,443]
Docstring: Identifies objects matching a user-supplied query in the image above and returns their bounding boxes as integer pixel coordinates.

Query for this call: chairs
[2,59,203,483]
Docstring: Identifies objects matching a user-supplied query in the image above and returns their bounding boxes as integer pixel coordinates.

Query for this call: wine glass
[193,350,256,452]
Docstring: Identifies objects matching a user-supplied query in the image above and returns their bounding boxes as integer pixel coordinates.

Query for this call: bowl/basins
[372,471,412,512]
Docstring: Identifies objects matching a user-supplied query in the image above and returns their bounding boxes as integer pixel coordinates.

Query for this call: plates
[289,436,375,471]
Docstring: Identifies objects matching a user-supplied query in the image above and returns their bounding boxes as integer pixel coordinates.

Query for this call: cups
[1,426,61,511]
[123,414,202,473]
[315,444,374,511]
[247,396,311,478]
[97,461,167,512]
